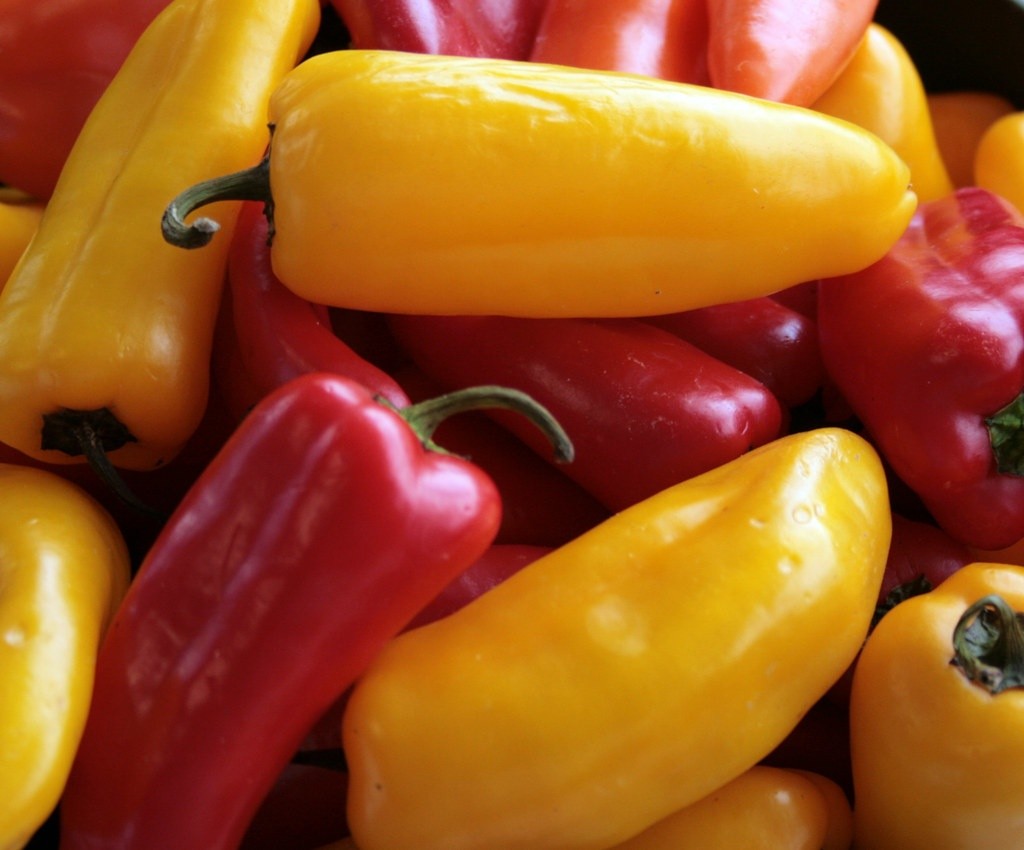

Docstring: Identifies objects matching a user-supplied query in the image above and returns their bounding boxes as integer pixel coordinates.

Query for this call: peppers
[1,0,1024,850]
